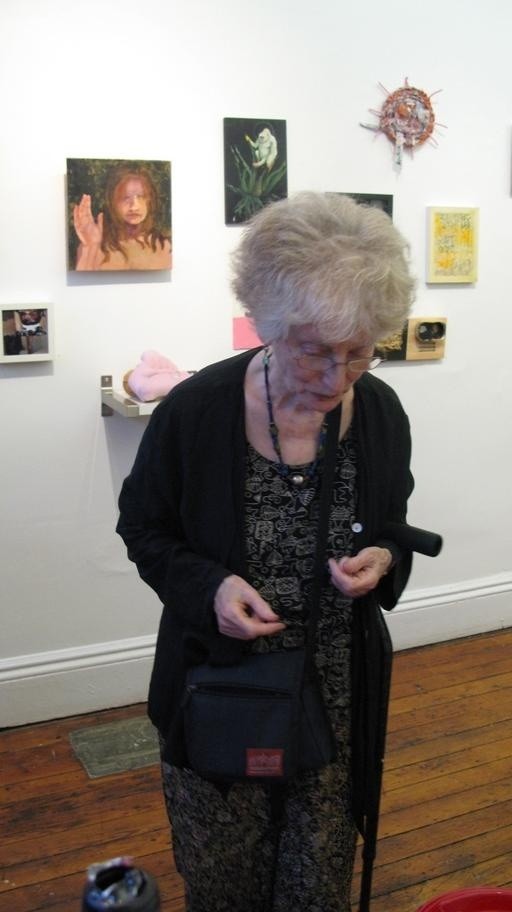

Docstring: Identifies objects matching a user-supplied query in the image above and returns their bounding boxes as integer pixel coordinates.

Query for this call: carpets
[66,710,163,779]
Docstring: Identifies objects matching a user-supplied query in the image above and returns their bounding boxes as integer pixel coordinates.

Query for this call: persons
[111,191,419,910]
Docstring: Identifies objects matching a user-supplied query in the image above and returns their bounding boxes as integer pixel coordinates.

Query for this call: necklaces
[258,346,335,491]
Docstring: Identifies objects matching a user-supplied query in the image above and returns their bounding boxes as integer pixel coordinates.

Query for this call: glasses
[283,337,388,373]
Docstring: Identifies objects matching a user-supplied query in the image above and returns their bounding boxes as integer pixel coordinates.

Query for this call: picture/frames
[1,303,56,364]
[427,204,481,284]
